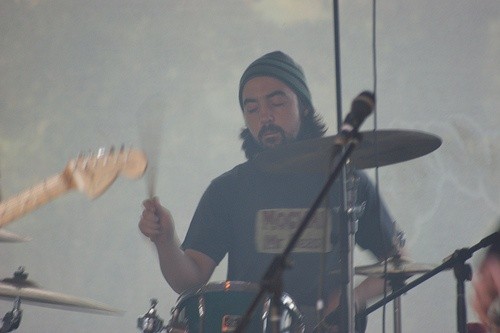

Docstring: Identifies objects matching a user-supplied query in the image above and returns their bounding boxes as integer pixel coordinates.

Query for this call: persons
[138,51,413,333]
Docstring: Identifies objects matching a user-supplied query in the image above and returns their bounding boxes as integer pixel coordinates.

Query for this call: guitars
[0,142,148,229]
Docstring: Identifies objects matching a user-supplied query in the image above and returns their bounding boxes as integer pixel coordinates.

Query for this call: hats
[239,51,311,110]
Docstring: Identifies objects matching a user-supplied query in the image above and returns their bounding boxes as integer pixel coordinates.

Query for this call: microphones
[329,91,375,159]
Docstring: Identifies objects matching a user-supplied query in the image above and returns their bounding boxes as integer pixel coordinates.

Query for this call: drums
[169,280,306,333]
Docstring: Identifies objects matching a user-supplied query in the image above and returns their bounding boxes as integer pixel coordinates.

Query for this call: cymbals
[0,278,125,316]
[0,229,32,242]
[354,257,454,279]
[253,130,442,172]
